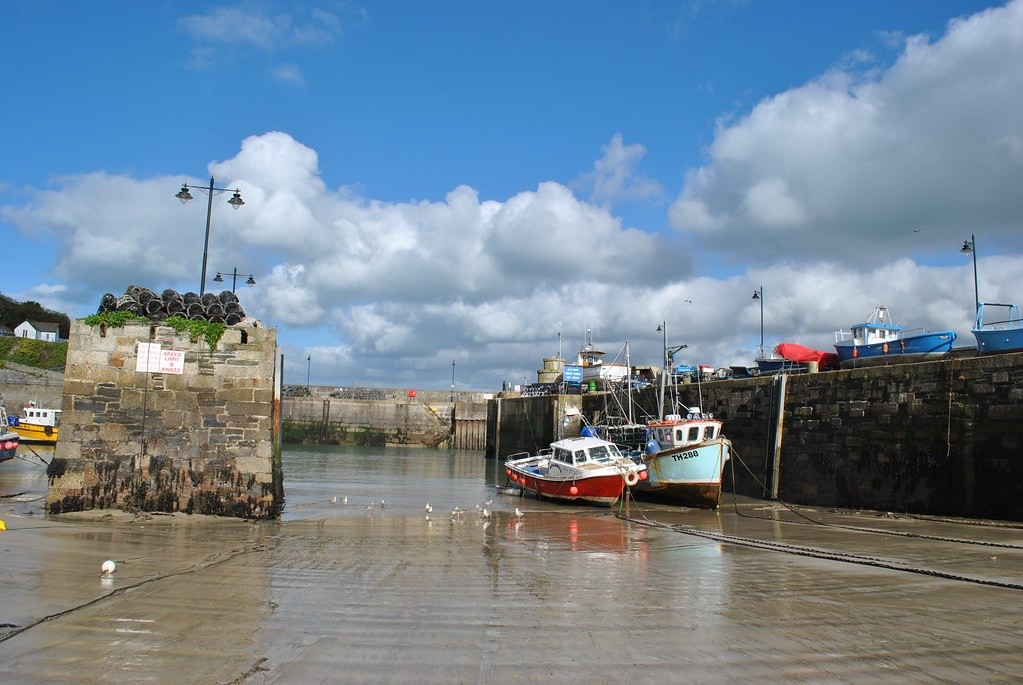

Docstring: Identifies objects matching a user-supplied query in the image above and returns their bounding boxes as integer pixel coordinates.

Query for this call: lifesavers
[624,470,639,486]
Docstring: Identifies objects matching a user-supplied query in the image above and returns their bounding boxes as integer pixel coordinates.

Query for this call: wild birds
[329,495,525,519]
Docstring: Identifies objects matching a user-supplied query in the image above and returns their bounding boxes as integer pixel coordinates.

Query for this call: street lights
[307,355,311,384]
[174,173,245,296]
[961,233,980,313]
[655,320,668,370]
[211,266,256,295]
[752,286,764,345]
[450,361,455,390]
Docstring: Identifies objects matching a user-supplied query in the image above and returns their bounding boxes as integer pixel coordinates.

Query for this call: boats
[833,304,958,369]
[503,411,645,510]
[970,302,1023,353]
[12,395,59,445]
[1,404,20,464]
[581,371,734,509]
[755,345,841,376]
